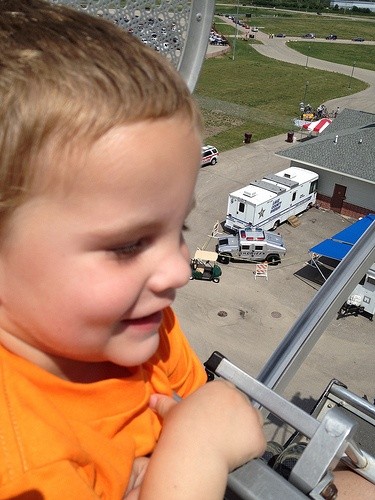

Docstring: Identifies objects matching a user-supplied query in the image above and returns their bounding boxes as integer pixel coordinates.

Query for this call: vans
[200,145,219,165]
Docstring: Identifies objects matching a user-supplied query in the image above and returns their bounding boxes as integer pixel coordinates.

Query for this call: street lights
[348,61,356,88]
[303,81,309,105]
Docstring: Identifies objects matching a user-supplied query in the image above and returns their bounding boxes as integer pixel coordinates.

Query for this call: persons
[302,104,339,118]
[0,0,375,500]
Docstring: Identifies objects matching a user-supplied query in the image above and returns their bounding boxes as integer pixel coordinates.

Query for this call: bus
[225,166,319,233]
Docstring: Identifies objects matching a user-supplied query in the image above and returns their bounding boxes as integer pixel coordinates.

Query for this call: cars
[352,37,364,42]
[276,33,286,37]
[214,12,258,32]
[326,34,337,40]
[304,33,316,38]
[218,228,286,265]
